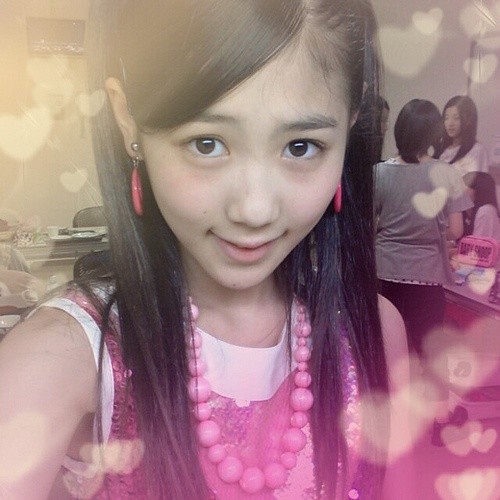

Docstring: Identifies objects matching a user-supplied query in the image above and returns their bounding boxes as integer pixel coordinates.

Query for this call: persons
[0,1,419,498]
[456,169,500,274]
[365,97,464,355]
[424,93,487,176]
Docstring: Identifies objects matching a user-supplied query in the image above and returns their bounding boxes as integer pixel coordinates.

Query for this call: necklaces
[149,278,318,493]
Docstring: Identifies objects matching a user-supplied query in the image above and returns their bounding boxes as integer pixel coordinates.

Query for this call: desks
[441,255,499,422]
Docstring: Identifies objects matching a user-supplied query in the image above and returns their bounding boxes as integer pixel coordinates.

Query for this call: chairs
[73,205,108,228]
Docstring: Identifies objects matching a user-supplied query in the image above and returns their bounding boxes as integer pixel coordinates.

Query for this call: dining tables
[0,225,112,267]
